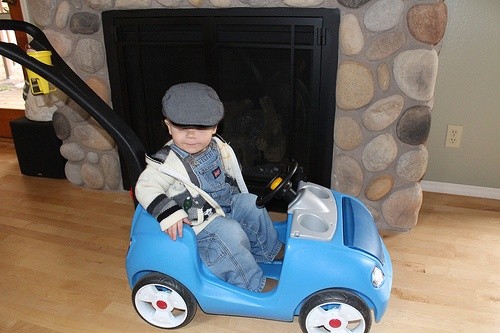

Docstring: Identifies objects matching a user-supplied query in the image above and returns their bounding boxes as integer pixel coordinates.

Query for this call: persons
[135,82,287,295]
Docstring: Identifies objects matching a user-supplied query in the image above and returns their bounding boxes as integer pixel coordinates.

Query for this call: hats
[162,82,225,126]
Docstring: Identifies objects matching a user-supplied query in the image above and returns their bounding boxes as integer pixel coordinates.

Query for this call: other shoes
[262,241,286,292]
